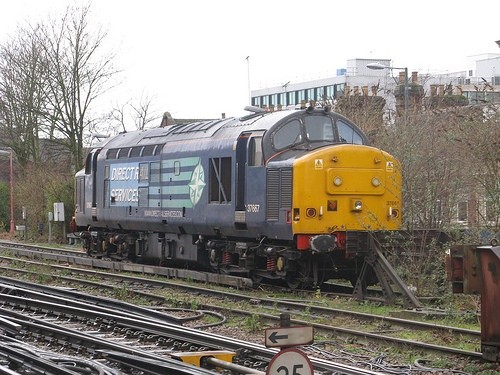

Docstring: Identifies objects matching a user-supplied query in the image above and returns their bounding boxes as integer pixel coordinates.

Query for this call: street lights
[367,62,408,112]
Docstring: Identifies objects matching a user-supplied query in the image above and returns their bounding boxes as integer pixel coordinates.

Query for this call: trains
[72,101,402,292]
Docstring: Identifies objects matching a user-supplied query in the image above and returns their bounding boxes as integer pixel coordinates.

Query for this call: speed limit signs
[265,348,314,375]
